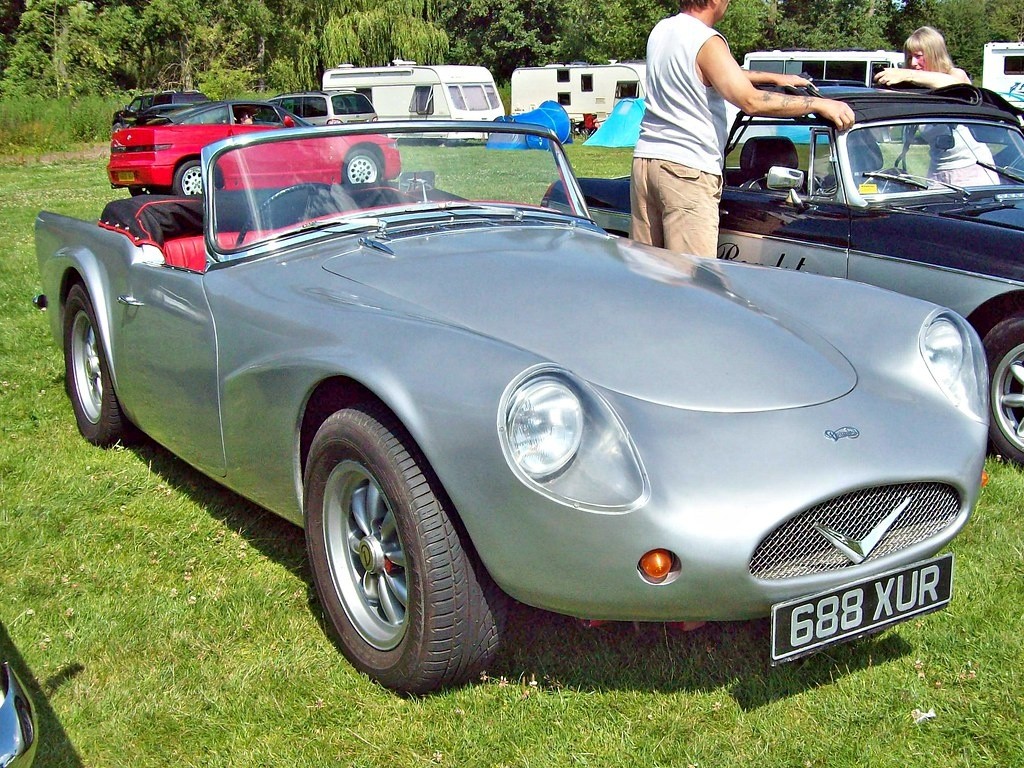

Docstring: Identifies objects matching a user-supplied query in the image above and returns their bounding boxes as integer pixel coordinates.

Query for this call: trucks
[322,60,505,139]
[510,60,647,133]
[982,41,1024,127]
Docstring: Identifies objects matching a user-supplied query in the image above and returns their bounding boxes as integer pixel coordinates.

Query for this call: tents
[585,98,646,147]
[487,100,571,150]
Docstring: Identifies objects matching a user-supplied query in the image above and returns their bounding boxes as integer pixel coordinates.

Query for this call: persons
[237,108,253,125]
[874,27,1000,186]
[631,0,855,261]
[584,114,598,136]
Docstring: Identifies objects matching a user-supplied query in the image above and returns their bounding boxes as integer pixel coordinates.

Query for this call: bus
[743,49,905,89]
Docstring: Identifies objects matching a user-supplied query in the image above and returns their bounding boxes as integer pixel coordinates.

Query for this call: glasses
[243,113,250,119]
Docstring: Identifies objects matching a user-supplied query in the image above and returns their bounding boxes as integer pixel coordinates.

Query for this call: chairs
[739,136,810,196]
[825,128,907,194]
[161,228,267,272]
[582,114,597,139]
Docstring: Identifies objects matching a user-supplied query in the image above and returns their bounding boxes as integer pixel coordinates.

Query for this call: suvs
[113,90,209,131]
[253,90,378,126]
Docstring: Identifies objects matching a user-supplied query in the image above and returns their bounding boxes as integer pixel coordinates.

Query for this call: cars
[543,86,1024,472]
[106,101,314,196]
[129,104,193,125]
[34,121,989,694]
